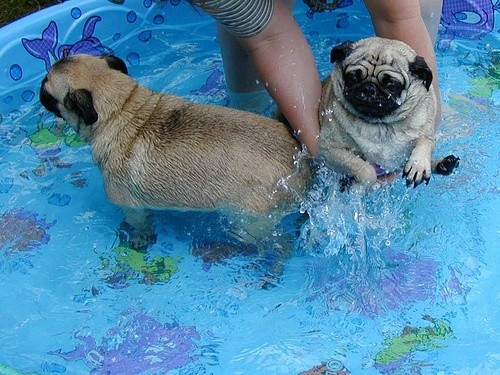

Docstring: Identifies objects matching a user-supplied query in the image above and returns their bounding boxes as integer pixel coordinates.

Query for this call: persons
[190,0,441,191]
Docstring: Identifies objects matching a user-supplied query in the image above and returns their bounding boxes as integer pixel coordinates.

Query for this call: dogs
[37,51,311,211]
[271,35,459,188]
